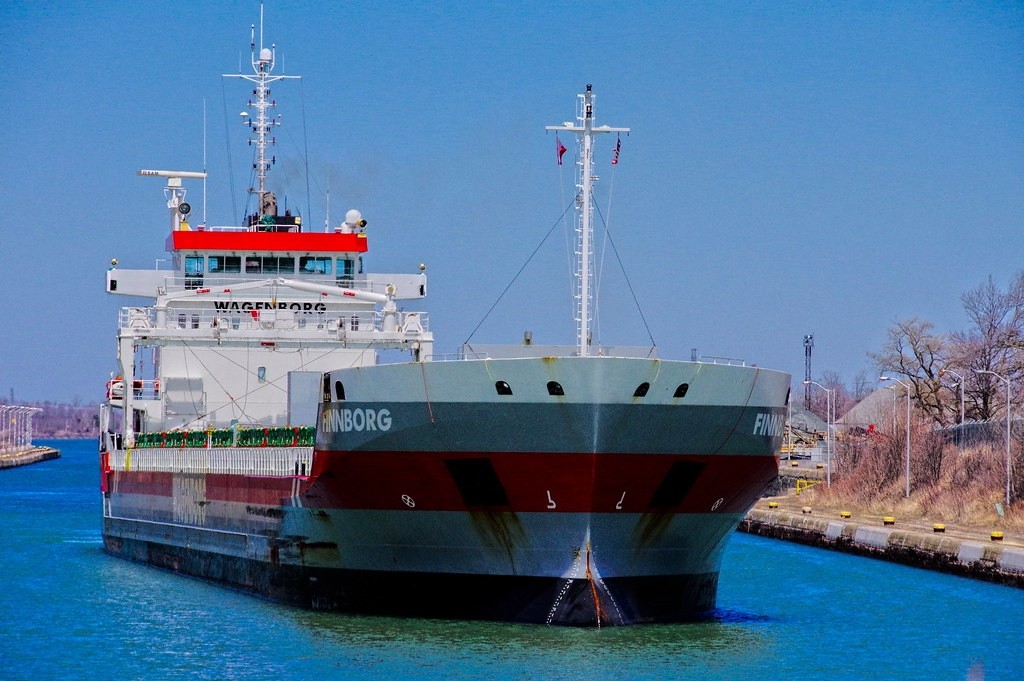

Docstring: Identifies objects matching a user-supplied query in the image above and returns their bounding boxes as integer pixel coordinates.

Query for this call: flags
[611,136,622,166]
[556,132,568,166]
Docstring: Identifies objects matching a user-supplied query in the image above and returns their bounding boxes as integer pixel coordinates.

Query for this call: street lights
[942,370,964,446]
[884,386,896,439]
[880,376,909,498]
[803,381,830,490]
[975,370,1010,506]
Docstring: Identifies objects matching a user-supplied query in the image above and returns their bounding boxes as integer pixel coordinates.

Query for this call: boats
[99,1,791,626]
